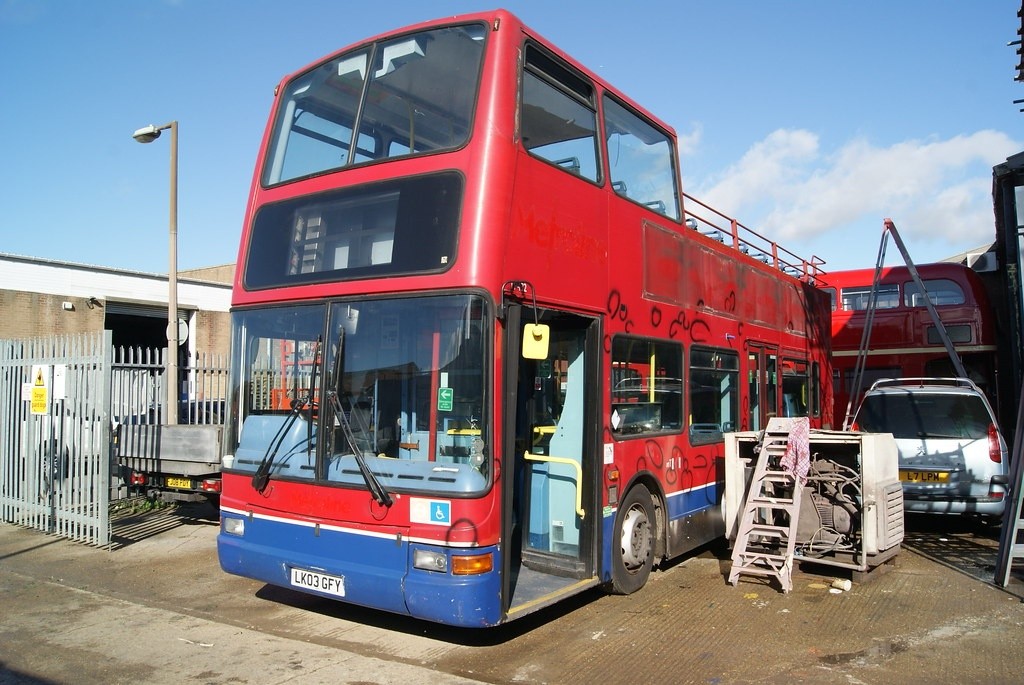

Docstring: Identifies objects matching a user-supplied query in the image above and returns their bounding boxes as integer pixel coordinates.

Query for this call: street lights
[132,120,179,424]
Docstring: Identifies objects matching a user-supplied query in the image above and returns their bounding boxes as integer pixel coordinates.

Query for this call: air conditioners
[966,252,997,272]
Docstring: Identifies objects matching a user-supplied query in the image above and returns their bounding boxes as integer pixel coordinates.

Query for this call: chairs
[348,396,389,449]
[559,158,801,280]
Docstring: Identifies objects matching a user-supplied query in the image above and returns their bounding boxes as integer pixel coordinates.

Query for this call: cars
[21,399,137,479]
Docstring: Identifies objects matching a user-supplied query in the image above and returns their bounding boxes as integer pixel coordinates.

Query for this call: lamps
[89,296,96,302]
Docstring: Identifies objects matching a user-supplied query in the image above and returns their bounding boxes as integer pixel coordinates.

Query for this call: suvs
[849,378,1011,530]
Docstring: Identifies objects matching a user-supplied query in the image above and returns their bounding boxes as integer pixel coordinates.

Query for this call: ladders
[994,379,1024,590]
[841,216,989,434]
[728,417,810,593]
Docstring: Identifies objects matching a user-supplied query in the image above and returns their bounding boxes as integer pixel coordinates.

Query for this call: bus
[799,263,1002,435]
[215,7,830,629]
